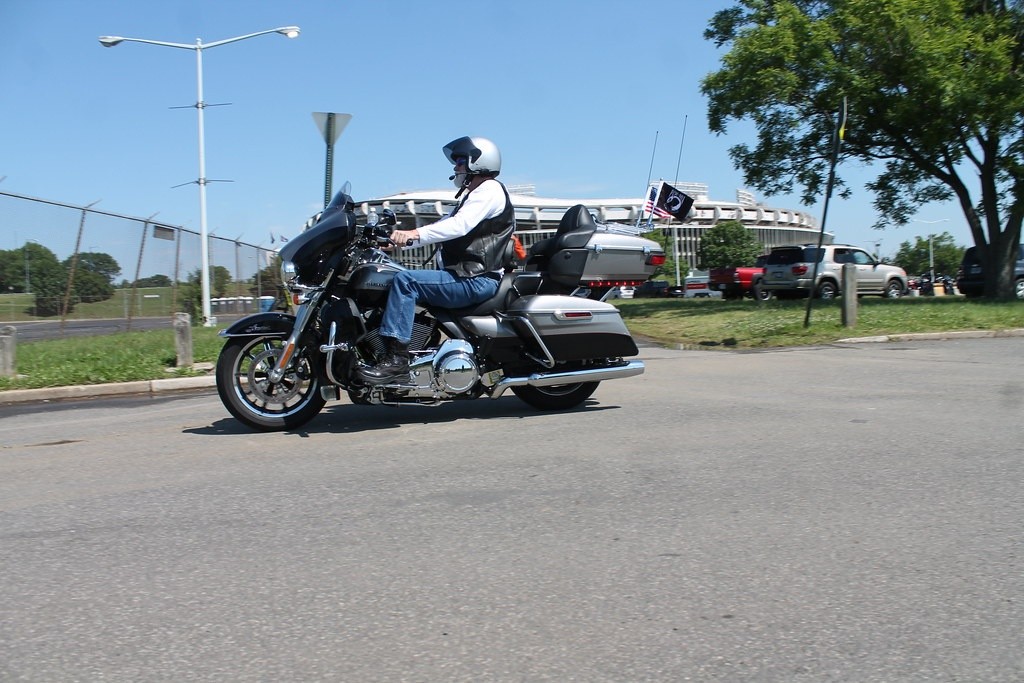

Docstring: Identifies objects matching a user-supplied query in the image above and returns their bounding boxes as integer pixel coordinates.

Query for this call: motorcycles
[214,114,697,434]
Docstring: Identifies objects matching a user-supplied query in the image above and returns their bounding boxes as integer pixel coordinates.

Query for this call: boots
[355,351,411,385]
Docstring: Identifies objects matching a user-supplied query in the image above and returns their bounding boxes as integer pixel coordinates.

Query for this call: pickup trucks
[708,253,776,301]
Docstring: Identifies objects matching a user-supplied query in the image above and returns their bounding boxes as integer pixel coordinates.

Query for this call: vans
[633,280,669,298]
[957,242,1024,300]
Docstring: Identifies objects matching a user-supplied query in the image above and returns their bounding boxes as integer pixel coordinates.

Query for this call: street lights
[95,24,308,327]
[910,219,948,284]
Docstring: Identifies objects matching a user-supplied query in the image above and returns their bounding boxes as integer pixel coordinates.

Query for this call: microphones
[449,173,465,180]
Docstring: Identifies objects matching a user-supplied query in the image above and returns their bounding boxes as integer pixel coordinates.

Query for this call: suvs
[769,242,909,306]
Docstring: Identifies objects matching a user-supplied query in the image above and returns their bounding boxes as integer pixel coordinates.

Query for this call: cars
[906,272,955,298]
[669,284,687,297]
[607,283,634,300]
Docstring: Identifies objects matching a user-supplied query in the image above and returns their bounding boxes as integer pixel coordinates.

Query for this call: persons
[354,136,517,384]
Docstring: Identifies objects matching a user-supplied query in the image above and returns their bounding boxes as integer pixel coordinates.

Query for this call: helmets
[443,136,501,178]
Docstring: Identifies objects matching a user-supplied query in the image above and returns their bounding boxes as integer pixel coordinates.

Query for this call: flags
[641,181,694,223]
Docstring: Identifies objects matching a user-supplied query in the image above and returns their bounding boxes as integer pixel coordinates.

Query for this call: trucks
[684,275,723,301]
